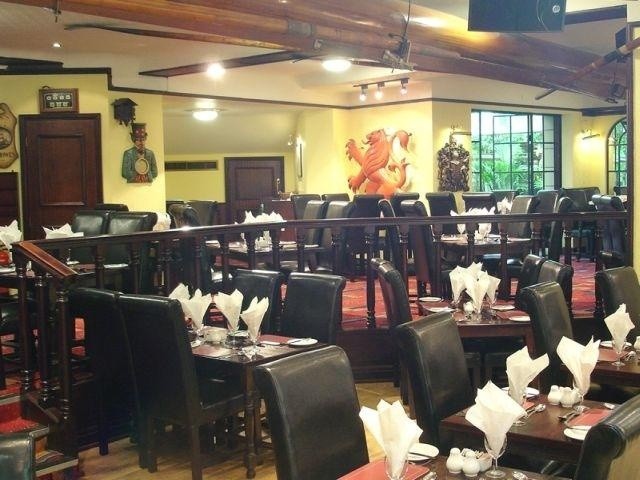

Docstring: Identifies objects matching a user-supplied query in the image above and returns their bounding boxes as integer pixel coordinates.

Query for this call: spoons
[626,351,636,362]
[422,472,437,480]
[557,407,583,423]
[603,402,617,410]
[511,470,529,480]
[524,403,547,420]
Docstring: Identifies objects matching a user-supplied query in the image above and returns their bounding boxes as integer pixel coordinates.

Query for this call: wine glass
[611,336,626,367]
[457,221,491,243]
[191,310,263,353]
[572,370,591,412]
[483,432,508,479]
[452,289,500,321]
[507,384,527,428]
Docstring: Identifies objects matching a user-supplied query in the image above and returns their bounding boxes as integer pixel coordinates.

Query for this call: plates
[205,238,320,252]
[562,424,590,441]
[285,338,318,348]
[400,442,439,462]
[0,260,129,274]
[442,235,528,245]
[499,385,540,398]
[418,295,531,324]
[598,340,632,349]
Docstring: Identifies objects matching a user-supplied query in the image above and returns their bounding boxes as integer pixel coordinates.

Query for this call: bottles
[445,447,480,478]
[547,385,573,408]
[633,335,640,350]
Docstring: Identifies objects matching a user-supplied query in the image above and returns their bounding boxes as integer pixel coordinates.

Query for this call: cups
[382,452,409,480]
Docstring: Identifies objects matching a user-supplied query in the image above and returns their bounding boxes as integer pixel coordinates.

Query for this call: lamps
[359,78,408,101]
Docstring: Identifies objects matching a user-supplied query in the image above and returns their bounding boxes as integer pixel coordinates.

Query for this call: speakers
[614,26,627,50]
[467,0,568,32]
[612,84,625,98]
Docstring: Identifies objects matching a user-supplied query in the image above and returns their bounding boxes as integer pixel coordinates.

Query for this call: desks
[444,376,623,478]
[563,340,640,402]
[192,325,327,478]
[348,448,550,480]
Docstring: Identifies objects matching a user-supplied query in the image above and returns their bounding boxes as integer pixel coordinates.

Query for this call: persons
[440,149,468,191]
[122,123,157,183]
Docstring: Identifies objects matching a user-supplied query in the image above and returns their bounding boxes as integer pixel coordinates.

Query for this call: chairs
[591,264,640,345]
[255,345,370,479]
[289,187,632,316]
[69,283,213,456]
[118,294,255,479]
[248,271,348,465]
[519,279,582,390]
[577,396,640,480]
[394,312,473,457]
[222,267,286,448]
[3,185,288,342]
